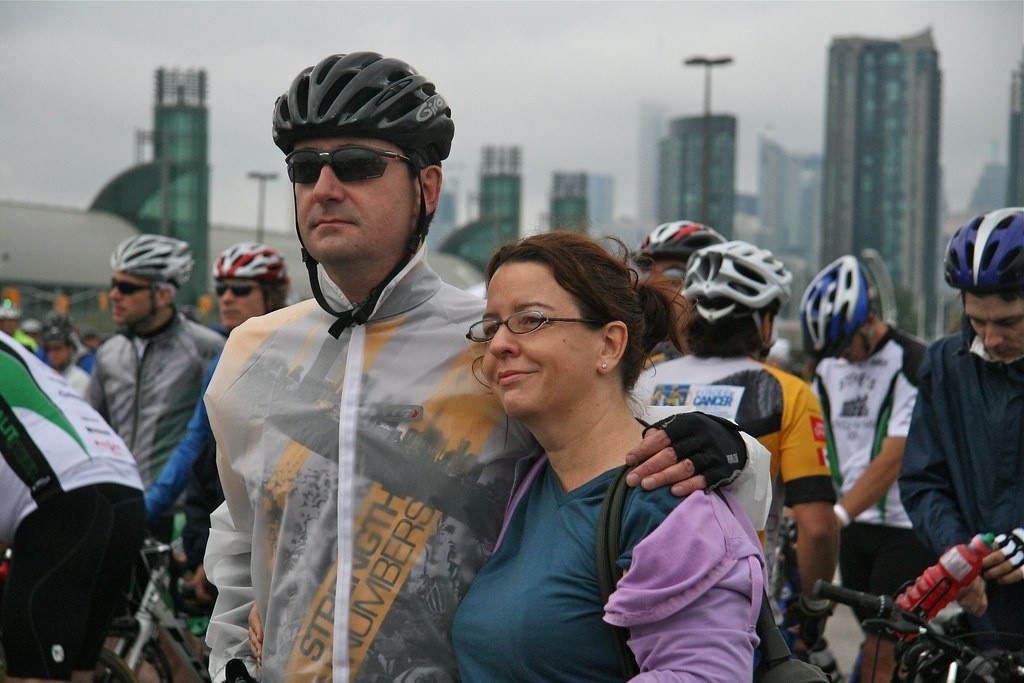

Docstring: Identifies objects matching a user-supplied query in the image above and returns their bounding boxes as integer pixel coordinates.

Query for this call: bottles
[895,532,996,640]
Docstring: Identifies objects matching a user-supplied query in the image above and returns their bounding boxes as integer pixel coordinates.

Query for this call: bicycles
[92,528,218,683]
[810,579,1024,683]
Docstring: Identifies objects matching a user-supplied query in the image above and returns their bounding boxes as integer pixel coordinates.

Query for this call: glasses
[641,265,689,286]
[284,144,426,184]
[212,281,262,299]
[466,307,597,348]
[110,277,160,295]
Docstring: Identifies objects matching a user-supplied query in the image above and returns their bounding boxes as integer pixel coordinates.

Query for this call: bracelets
[832,504,851,529]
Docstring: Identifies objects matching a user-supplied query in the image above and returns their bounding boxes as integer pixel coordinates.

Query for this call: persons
[449,229,766,683]
[0,206,1024,683]
[202,52,773,683]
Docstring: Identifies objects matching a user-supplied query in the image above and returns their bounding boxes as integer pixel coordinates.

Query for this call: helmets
[211,243,293,285]
[634,220,728,267]
[111,233,194,290]
[943,206,1023,294]
[799,254,871,358]
[683,241,794,321]
[272,52,454,167]
[1,297,82,360]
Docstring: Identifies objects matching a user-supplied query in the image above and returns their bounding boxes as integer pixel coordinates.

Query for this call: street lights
[249,172,279,245]
[684,57,733,228]
